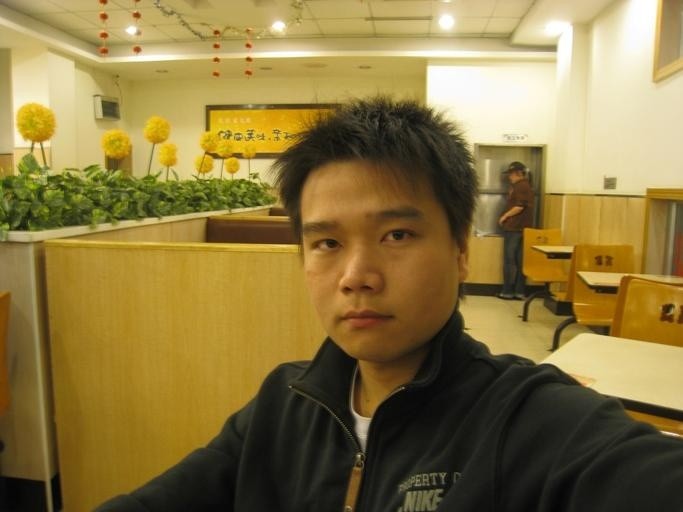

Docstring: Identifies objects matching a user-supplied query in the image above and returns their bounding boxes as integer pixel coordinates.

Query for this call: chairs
[519,227,683,437]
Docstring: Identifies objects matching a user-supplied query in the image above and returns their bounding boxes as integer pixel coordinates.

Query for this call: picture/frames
[205,103,341,159]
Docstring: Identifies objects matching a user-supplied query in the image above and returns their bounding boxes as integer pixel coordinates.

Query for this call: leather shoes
[495,293,522,299]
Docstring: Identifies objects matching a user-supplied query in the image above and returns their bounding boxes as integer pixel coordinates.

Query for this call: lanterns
[212,27,221,80]
[130,0,143,56]
[243,27,254,80]
[96,0,108,59]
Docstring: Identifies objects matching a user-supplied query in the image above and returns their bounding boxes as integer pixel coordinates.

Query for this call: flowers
[0,104,278,242]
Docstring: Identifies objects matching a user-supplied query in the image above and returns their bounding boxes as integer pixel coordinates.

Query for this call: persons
[91,88,683,510]
[492,160,535,301]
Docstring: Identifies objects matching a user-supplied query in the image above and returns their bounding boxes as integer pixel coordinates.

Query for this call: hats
[502,162,525,173]
